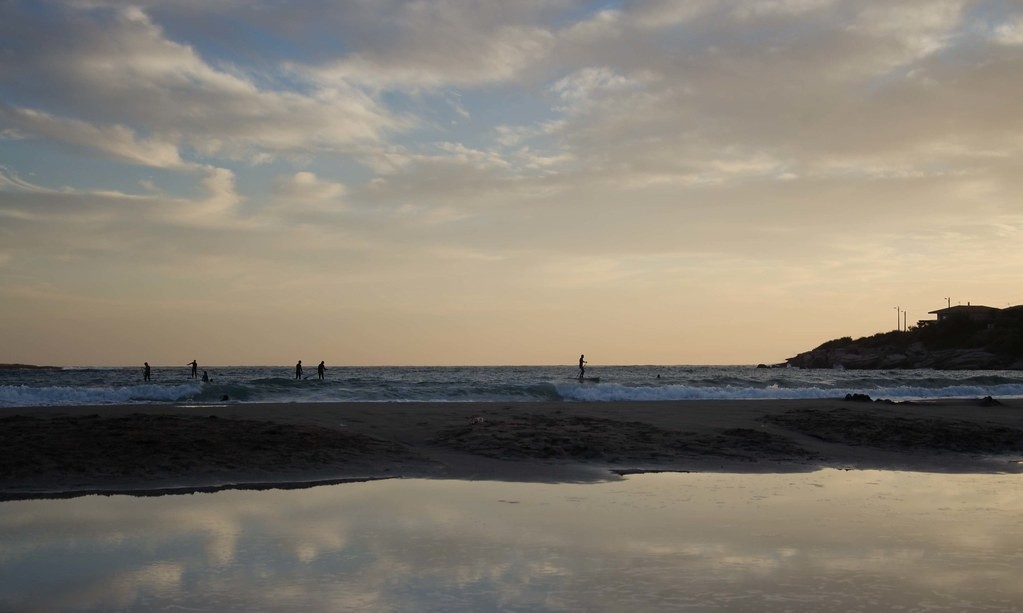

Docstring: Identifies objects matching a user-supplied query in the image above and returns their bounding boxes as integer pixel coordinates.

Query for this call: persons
[295,360,302,380]
[318,360,326,380]
[143,362,150,382]
[187,360,197,378]
[579,355,588,378]
[202,371,209,383]
